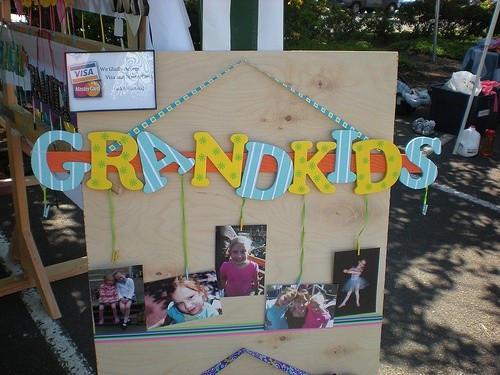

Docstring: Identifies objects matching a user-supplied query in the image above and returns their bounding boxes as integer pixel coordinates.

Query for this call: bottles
[457,125,481,157]
[481,128,496,157]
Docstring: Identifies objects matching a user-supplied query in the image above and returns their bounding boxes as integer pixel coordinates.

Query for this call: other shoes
[413,118,424,133]
[121,322,127,330]
[98,319,104,325]
[115,317,119,325]
[422,120,434,135]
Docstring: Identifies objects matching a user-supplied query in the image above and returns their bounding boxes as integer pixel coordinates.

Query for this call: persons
[162,275,219,326]
[337,259,369,308]
[98,270,134,330]
[219,236,260,297]
[266,287,331,329]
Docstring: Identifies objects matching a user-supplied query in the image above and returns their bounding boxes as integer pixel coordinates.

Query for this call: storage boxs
[427,84,496,136]
